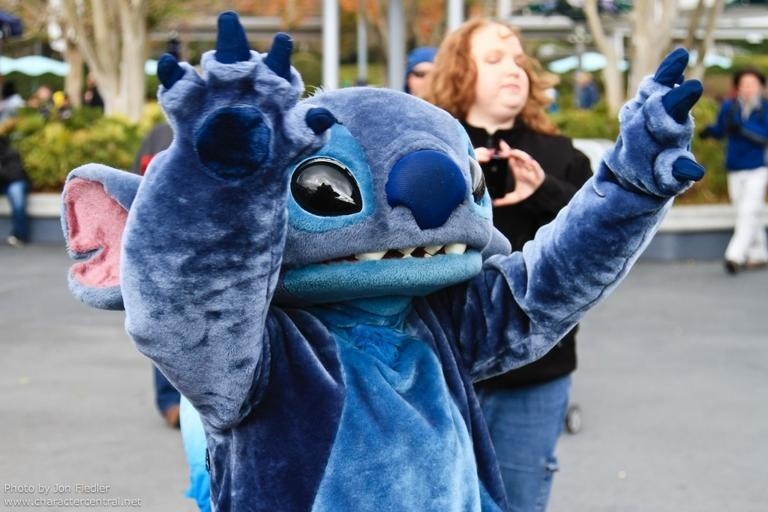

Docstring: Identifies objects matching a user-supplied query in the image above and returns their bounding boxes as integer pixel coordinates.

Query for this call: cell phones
[481,156,508,198]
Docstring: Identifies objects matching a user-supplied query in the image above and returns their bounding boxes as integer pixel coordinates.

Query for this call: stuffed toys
[59,10,707,512]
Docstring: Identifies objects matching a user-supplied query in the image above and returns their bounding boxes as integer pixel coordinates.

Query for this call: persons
[422,17,594,512]
[402,47,441,101]
[697,68,767,273]
[1,135,35,247]
[1,69,104,122]
